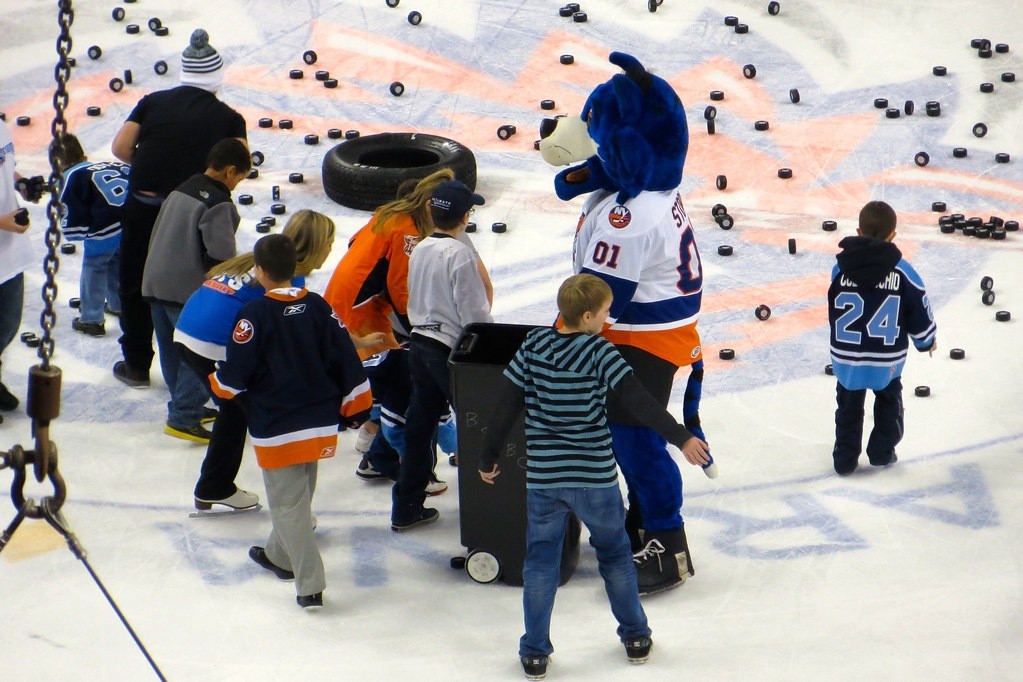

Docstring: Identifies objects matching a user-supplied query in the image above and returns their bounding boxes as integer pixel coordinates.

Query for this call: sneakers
[355,424,378,453]
[189,487,263,518]
[425,479,448,497]
[104,301,121,316]
[200,406,219,423]
[355,456,391,482]
[72,317,106,337]
[391,507,439,533]
[164,420,213,444]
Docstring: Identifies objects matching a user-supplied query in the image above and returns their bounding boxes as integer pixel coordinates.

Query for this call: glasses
[465,208,475,216]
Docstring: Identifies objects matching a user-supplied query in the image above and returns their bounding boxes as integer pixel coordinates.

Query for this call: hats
[430,180,485,221]
[180,29,224,92]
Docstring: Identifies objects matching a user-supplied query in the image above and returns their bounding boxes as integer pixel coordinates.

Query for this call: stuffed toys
[538,52,716,592]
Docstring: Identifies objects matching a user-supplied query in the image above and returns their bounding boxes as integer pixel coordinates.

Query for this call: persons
[111,28,249,391]
[0,120,45,425]
[828,201,938,477]
[478,274,710,682]
[324,168,494,493]
[206,235,373,611]
[141,139,252,446]
[49,135,132,336]
[389,181,498,532]
[172,209,332,518]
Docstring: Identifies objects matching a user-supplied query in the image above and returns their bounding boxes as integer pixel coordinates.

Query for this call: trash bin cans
[447,321,581,589]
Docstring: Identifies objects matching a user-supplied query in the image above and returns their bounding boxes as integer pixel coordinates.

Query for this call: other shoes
[296,591,324,612]
[870,451,897,470]
[249,546,294,581]
[626,637,650,664]
[0,381,19,423]
[113,361,150,387]
[522,652,547,680]
